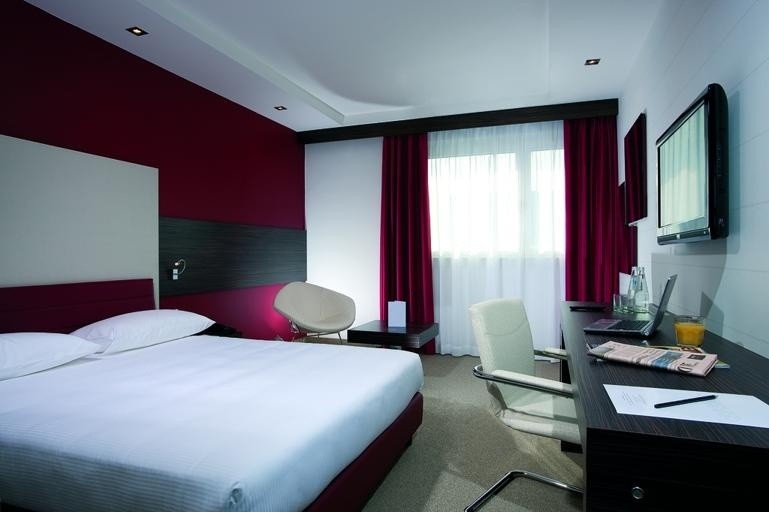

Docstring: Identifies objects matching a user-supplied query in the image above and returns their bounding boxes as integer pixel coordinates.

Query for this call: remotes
[568,304,605,313]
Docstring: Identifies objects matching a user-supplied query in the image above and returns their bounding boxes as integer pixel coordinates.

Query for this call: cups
[613,293,629,314]
[673,316,706,346]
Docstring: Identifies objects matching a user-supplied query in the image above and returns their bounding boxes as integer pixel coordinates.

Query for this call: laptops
[581,274,678,337]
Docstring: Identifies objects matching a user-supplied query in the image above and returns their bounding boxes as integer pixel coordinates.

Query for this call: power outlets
[172,268,180,280]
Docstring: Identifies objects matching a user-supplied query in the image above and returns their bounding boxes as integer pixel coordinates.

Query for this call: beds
[0,277,426,512]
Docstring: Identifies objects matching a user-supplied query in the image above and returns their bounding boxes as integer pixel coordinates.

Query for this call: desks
[558,300,769,512]
[347,318,440,353]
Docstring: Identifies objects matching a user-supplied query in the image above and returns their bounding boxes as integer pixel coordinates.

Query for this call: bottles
[633,267,650,313]
[627,267,638,313]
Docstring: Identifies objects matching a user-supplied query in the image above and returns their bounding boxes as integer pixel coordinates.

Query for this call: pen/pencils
[654,395,721,409]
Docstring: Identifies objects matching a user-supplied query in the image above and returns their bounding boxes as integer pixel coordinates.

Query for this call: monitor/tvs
[656,82,728,248]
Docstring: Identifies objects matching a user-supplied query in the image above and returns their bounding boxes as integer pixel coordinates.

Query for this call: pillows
[68,308,217,357]
[0,331,102,381]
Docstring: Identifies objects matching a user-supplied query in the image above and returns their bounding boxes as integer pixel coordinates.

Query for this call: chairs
[464,297,584,512]
[273,281,356,344]
